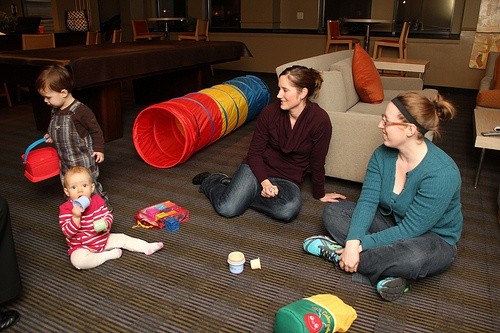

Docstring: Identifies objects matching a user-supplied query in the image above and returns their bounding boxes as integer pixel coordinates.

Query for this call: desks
[147,17,183,41]
[344,18,392,52]
[0,41,255,145]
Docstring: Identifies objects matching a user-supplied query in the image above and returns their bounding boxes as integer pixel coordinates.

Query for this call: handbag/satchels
[131,201,191,230]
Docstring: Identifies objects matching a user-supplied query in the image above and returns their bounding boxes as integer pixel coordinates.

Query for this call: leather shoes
[0,309,20,333]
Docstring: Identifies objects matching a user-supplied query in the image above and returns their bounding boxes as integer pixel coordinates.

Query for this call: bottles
[227,252,246,274]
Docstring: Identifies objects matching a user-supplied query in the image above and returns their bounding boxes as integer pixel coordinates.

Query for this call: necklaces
[289,110,298,119]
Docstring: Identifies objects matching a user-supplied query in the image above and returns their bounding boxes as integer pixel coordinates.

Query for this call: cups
[250,257,262,270]
[73,196,90,212]
[93,219,107,233]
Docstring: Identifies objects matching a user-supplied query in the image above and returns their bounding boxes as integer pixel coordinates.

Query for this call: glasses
[381,113,409,127]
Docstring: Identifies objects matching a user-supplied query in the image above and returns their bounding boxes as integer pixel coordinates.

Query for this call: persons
[0,198,23,330]
[302,92,464,301]
[192,65,346,222]
[37,64,114,214]
[58,166,163,270]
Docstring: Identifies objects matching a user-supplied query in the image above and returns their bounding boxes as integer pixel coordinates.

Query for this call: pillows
[353,42,384,104]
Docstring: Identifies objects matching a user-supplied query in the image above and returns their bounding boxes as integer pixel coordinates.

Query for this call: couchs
[276,49,424,184]
[475,51,500,110]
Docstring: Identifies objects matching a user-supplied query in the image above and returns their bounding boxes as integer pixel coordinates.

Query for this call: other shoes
[192,171,224,185]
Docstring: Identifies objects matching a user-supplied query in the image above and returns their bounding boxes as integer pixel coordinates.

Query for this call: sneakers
[302,236,345,274]
[377,277,414,302]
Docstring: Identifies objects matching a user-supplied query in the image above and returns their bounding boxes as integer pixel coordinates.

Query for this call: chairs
[132,18,164,41]
[324,19,361,55]
[0,28,125,109]
[373,21,411,77]
[177,17,210,42]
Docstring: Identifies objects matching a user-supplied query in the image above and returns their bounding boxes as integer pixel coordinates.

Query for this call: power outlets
[296,12,303,20]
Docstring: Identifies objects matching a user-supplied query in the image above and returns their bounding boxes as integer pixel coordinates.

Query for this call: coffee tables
[472,109,500,189]
[370,56,432,80]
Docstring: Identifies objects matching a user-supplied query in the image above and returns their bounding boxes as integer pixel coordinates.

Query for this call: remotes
[480,130,500,136]
